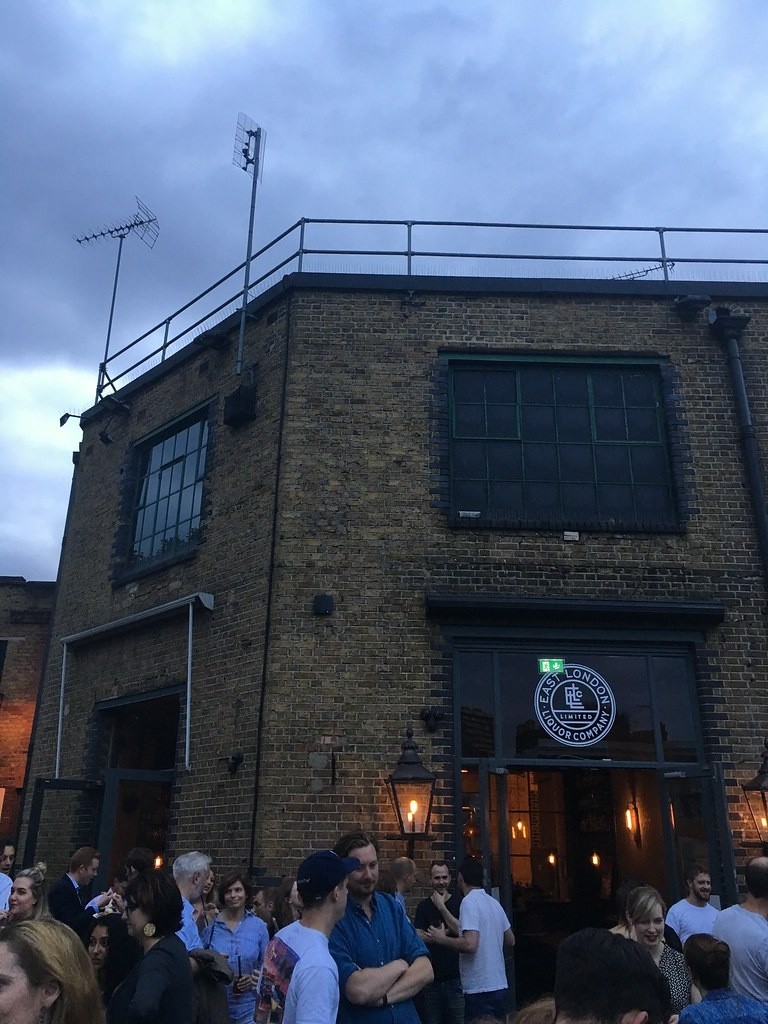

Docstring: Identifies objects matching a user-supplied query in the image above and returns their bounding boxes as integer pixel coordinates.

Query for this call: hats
[297,849,359,900]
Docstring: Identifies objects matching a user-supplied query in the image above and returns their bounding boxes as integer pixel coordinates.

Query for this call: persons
[0,833,768,1024]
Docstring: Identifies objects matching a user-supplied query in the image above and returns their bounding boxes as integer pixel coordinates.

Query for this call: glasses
[125,903,136,914]
